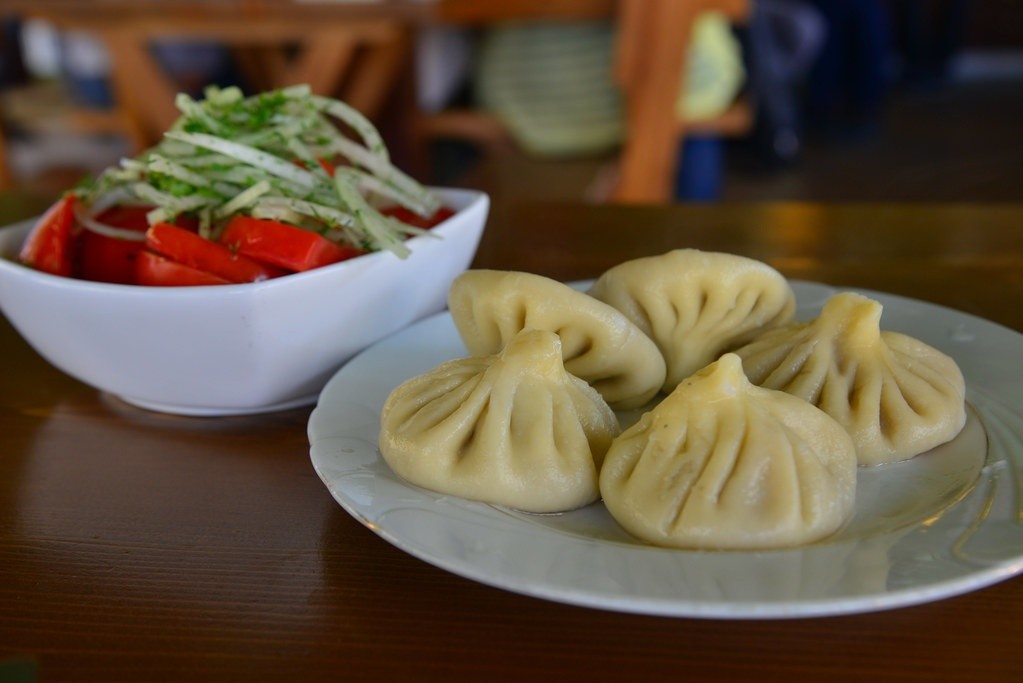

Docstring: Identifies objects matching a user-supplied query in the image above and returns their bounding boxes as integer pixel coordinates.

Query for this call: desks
[1,202,1023,683]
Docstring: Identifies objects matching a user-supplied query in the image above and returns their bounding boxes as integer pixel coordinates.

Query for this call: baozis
[373,244,969,551]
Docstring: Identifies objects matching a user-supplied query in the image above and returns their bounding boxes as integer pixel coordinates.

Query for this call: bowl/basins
[0,185,489,418]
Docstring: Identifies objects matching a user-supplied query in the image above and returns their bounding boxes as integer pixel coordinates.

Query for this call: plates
[305,279,1023,618]
